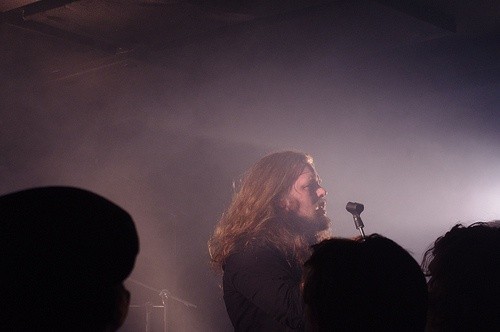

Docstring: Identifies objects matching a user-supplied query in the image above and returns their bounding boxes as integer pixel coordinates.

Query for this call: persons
[420,220,500,332]
[0,186,140,332]
[207,151,330,332]
[301,232,428,332]
